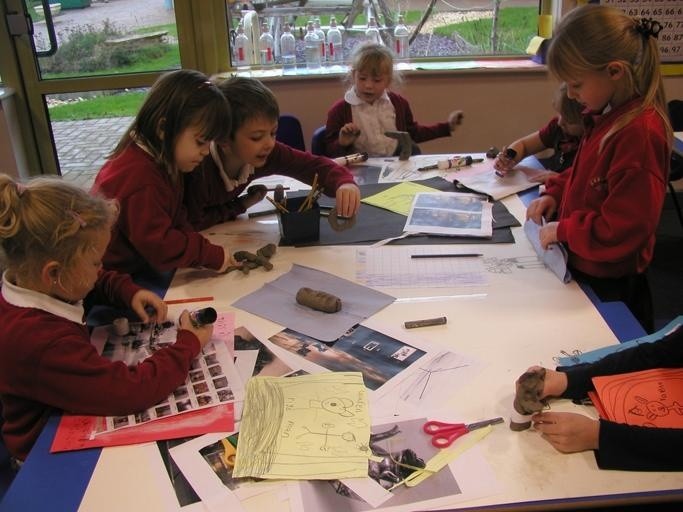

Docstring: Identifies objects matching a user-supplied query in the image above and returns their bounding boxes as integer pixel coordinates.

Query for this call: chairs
[278,115,336,162]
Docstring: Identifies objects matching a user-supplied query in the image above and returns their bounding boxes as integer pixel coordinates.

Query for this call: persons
[170,76,360,231]
[323,45,464,158]
[0,171,215,472]
[494,79,593,185]
[526,315,683,474]
[86,70,241,288]
[525,4,675,334]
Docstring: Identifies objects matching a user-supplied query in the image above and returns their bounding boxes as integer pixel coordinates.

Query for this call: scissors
[423,418,504,448]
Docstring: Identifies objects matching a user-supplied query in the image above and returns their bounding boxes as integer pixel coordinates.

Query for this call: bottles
[234,23,252,78]
[327,16,344,74]
[303,20,321,74]
[280,21,298,76]
[394,15,410,70]
[365,17,381,45]
[313,18,326,67]
[259,22,276,77]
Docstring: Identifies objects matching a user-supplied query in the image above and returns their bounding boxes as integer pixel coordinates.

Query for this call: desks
[2,152,681,512]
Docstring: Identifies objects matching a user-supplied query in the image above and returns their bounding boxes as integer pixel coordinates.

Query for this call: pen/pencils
[411,254,483,258]
[248,172,325,218]
[417,159,484,172]
[165,297,213,304]
